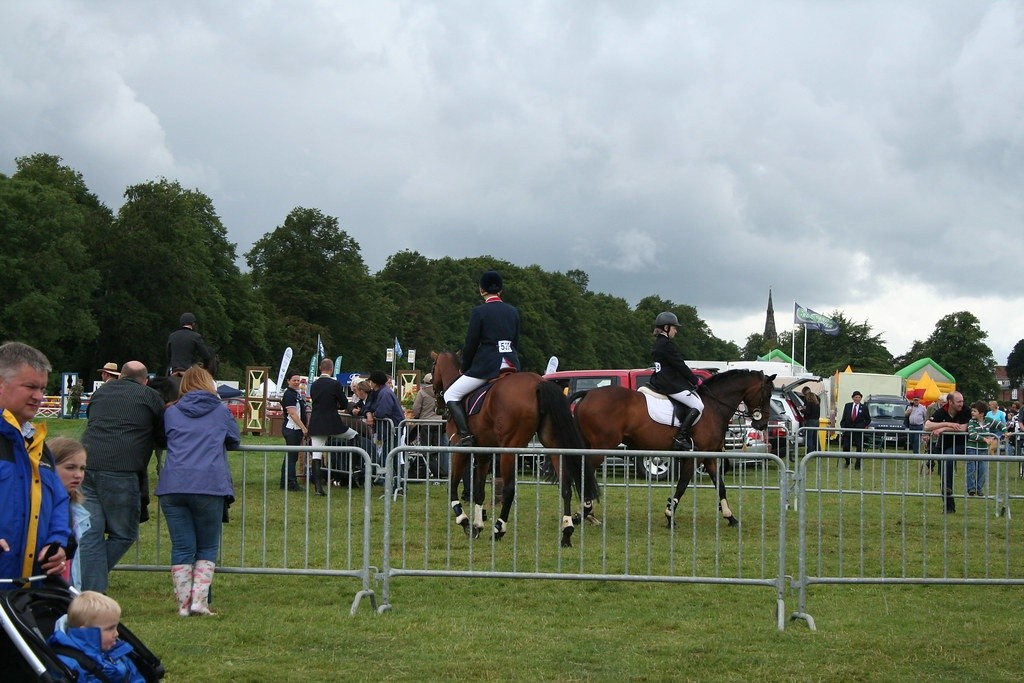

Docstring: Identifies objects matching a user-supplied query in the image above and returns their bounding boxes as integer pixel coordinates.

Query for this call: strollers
[1,537,166,683]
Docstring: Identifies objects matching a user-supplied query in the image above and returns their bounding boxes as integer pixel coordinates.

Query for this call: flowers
[401,392,416,408]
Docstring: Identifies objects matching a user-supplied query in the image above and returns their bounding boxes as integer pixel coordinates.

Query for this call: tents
[218,384,242,398]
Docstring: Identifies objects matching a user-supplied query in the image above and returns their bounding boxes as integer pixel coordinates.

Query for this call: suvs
[541,367,824,481]
[860,394,912,450]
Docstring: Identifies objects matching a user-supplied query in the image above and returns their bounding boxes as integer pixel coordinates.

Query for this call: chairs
[893,405,905,415]
[870,405,881,416]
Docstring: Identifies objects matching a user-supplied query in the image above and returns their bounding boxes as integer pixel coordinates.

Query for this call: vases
[405,409,415,419]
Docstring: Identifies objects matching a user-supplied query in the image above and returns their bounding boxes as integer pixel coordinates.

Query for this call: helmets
[655,312,683,327]
[180,313,197,325]
[480,271,502,294]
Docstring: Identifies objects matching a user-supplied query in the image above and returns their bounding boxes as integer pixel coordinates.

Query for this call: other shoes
[943,510,955,514]
[969,489,974,495]
[978,492,984,495]
[289,484,305,492]
[942,487,952,495]
[855,466,860,471]
[844,463,848,468]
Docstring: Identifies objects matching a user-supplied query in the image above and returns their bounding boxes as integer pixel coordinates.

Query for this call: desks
[265,415,285,435]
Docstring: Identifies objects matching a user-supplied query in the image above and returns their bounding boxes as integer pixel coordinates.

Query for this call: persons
[344,370,409,497]
[441,267,522,449]
[154,363,241,616]
[904,391,1011,514]
[77,360,166,595]
[412,373,449,479]
[650,307,705,451]
[0,342,147,683]
[984,400,1024,479]
[798,385,821,454]
[308,357,362,497]
[165,313,212,375]
[840,390,871,471]
[281,369,308,492]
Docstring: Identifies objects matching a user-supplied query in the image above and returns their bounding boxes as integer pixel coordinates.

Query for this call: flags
[794,303,828,334]
[806,309,840,336]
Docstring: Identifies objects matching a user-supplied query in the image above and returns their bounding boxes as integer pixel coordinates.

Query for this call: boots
[190,560,217,615]
[447,401,476,447]
[349,433,362,448]
[311,459,327,496]
[172,563,193,616]
[673,408,701,450]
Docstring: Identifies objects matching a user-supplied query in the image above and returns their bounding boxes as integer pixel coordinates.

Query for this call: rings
[60,562,66,566]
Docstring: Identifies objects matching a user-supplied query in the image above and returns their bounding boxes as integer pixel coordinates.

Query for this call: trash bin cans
[818,418,828,451]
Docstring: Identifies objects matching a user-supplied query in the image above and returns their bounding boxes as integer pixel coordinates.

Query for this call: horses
[427,347,601,549]
[568,368,777,524]
[147,373,183,476]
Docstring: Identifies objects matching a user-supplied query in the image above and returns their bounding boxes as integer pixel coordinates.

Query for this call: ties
[851,405,857,421]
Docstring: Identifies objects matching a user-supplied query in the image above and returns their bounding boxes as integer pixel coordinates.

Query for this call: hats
[423,373,433,384]
[97,362,122,375]
[851,391,862,398]
[367,371,388,385]
[913,396,920,400]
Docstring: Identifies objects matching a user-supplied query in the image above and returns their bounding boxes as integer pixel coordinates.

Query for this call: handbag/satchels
[904,416,909,428]
